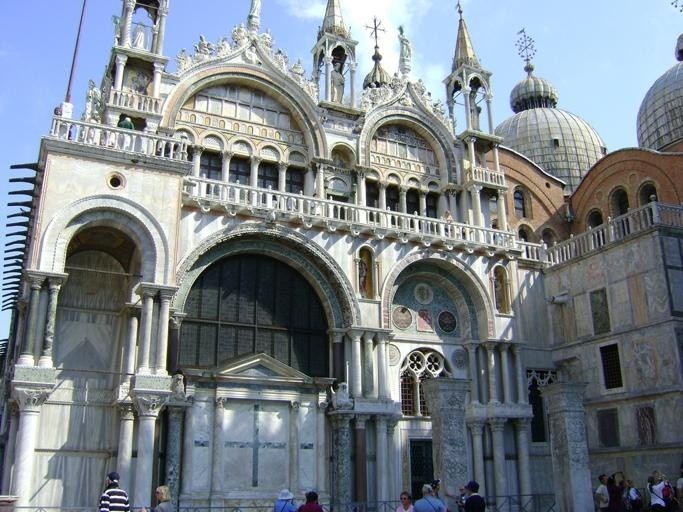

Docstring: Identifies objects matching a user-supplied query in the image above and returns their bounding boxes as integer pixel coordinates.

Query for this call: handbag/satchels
[661,497,672,505]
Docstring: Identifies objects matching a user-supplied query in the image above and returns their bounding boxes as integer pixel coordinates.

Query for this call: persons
[442,210,455,232]
[360,73,447,120]
[413,484,447,512]
[140,484,180,512]
[396,25,412,77]
[97,470,133,512]
[132,17,150,49]
[81,78,104,124]
[298,491,324,512]
[272,488,298,512]
[464,480,486,512]
[118,117,135,130]
[456,486,465,512]
[590,463,682,512]
[330,60,346,103]
[490,217,498,240]
[174,21,304,79]
[393,491,414,511]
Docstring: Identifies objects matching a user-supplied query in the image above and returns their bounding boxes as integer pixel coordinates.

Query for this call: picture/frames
[414,283,434,305]
[416,306,436,333]
[391,305,414,331]
[436,309,458,334]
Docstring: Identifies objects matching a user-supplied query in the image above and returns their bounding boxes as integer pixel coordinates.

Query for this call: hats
[464,481,479,492]
[432,479,440,485]
[277,489,294,500]
[107,472,120,481]
